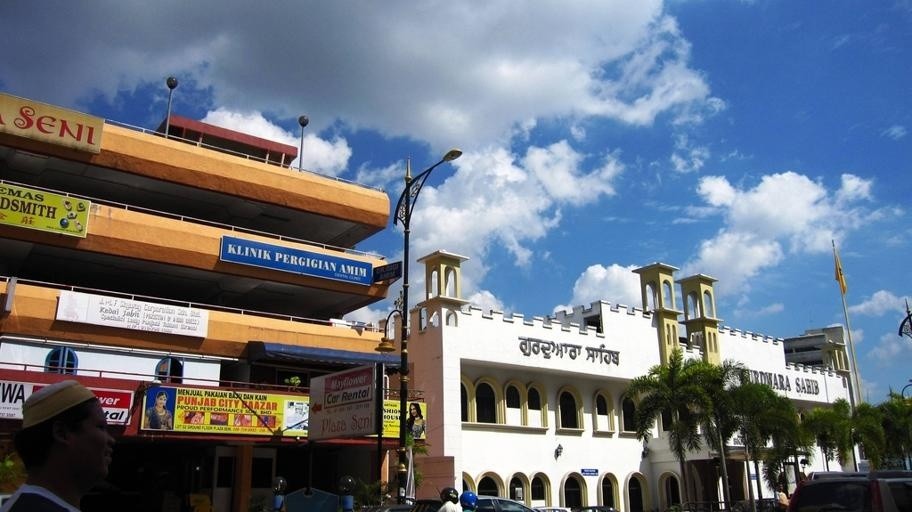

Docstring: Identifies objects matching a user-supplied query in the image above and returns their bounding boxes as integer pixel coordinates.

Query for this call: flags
[833,248,847,295]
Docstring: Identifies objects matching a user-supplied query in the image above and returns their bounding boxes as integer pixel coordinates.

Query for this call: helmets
[440,487,458,504]
[460,491,479,510]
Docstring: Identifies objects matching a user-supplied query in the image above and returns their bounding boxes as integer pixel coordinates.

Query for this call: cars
[787,471,912,512]
[380,495,619,512]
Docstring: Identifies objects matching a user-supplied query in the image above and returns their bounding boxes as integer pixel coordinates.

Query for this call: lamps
[556,444,564,456]
[642,447,650,458]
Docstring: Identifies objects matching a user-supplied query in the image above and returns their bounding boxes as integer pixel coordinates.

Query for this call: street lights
[375,148,462,504]
[165,76,178,139]
[299,115,309,170]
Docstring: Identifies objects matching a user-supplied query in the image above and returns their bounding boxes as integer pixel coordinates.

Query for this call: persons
[406,402,425,440]
[144,390,173,429]
[0,380,118,511]
[437,487,459,512]
[460,491,478,512]
[774,481,790,511]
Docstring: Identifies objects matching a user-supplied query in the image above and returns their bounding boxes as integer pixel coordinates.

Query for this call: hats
[22,378,97,430]
